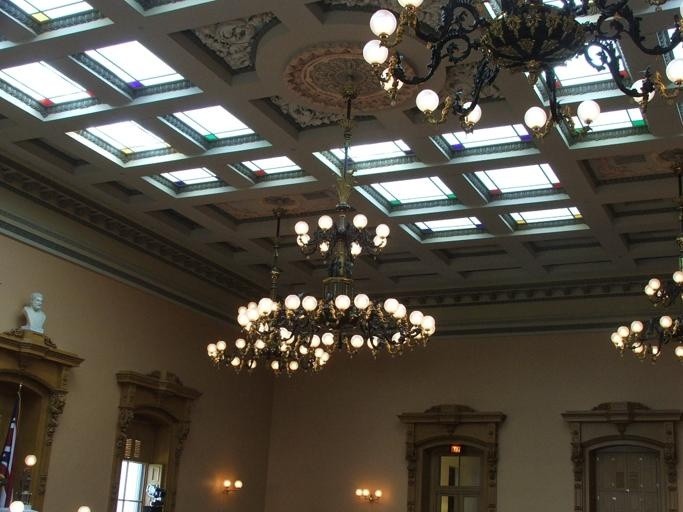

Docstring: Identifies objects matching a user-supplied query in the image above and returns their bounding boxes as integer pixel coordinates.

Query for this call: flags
[0,390,21,508]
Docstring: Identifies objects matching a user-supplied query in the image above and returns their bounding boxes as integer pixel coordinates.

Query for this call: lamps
[365,1,683,142]
[222,479,243,495]
[610,147,683,364]
[355,488,382,505]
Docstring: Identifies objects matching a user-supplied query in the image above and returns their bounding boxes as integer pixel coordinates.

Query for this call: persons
[17,291,47,336]
